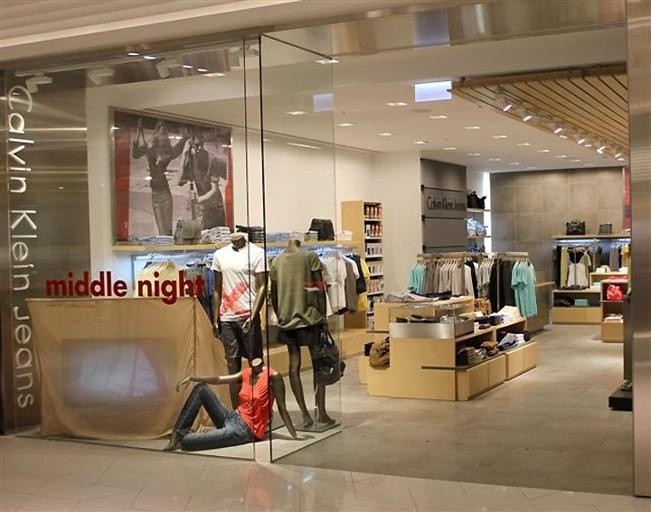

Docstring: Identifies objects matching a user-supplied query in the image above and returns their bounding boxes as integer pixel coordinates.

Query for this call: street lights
[469,242,486,252]
[311,217,336,241]
[467,218,488,237]
[314,328,347,386]
[468,190,488,209]
[565,218,587,236]
[599,223,614,236]
[173,218,205,245]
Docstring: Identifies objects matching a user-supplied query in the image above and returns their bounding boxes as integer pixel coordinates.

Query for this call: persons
[174,127,224,228]
[209,235,269,408]
[132,116,188,235]
[160,357,306,451]
[268,238,335,428]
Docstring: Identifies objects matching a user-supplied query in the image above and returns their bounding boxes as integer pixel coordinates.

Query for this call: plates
[466,207,491,239]
[361,296,536,401]
[340,200,383,328]
[526,272,629,342]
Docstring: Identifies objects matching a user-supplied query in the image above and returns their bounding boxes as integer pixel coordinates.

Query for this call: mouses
[566,245,589,259]
[143,254,177,276]
[610,238,631,249]
[479,256,532,270]
[267,245,358,262]
[415,255,464,272]
